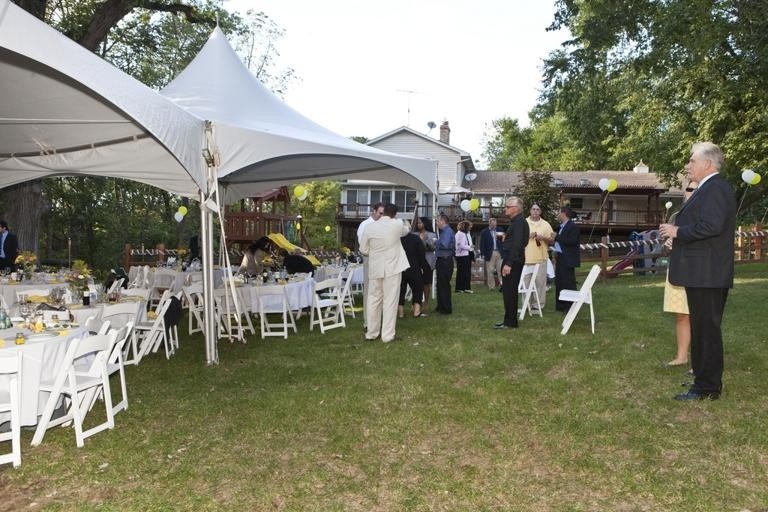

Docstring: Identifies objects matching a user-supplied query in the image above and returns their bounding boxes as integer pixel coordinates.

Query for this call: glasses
[685,187,696,192]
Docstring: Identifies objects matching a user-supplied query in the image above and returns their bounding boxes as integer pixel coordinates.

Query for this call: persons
[659,142,737,403]
[522,202,555,313]
[278,248,314,311]
[663,181,699,367]
[357,196,530,343]
[0,221,18,274]
[187,235,202,267]
[555,207,580,312]
[237,236,275,277]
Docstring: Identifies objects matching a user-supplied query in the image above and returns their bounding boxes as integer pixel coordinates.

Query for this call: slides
[269,232,322,268]
[606,251,638,277]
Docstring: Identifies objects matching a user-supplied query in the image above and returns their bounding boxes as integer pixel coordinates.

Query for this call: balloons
[297,190,307,201]
[296,223,301,230]
[741,169,754,184]
[607,178,617,192]
[325,226,330,232]
[665,201,673,209]
[751,173,761,185]
[599,178,610,192]
[294,186,305,197]
[179,206,187,217]
[174,212,183,223]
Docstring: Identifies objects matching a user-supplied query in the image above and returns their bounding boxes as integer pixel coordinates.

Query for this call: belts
[0,234,3,255]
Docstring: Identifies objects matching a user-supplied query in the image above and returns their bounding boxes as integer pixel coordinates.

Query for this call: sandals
[667,359,688,366]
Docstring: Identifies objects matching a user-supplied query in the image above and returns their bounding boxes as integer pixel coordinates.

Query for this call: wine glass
[1,263,11,278]
[19,293,35,328]
[49,286,64,305]
[92,282,124,304]
[39,265,70,279]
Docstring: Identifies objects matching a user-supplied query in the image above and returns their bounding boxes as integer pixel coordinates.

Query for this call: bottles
[241,266,288,282]
[534,231,541,247]
[83,280,91,306]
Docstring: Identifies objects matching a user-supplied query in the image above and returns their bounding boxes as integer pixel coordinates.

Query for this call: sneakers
[494,322,518,329]
[398,288,474,318]
[672,383,722,400]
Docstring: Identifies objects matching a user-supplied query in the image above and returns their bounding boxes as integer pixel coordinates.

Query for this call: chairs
[183,272,254,343]
[311,254,355,334]
[1,351,22,468]
[30,329,118,446]
[134,289,182,364]
[129,266,150,288]
[517,263,543,320]
[106,266,128,299]
[558,264,601,335]
[81,317,111,334]
[101,302,141,367]
[256,286,297,339]
[66,320,135,428]
[15,289,49,302]
